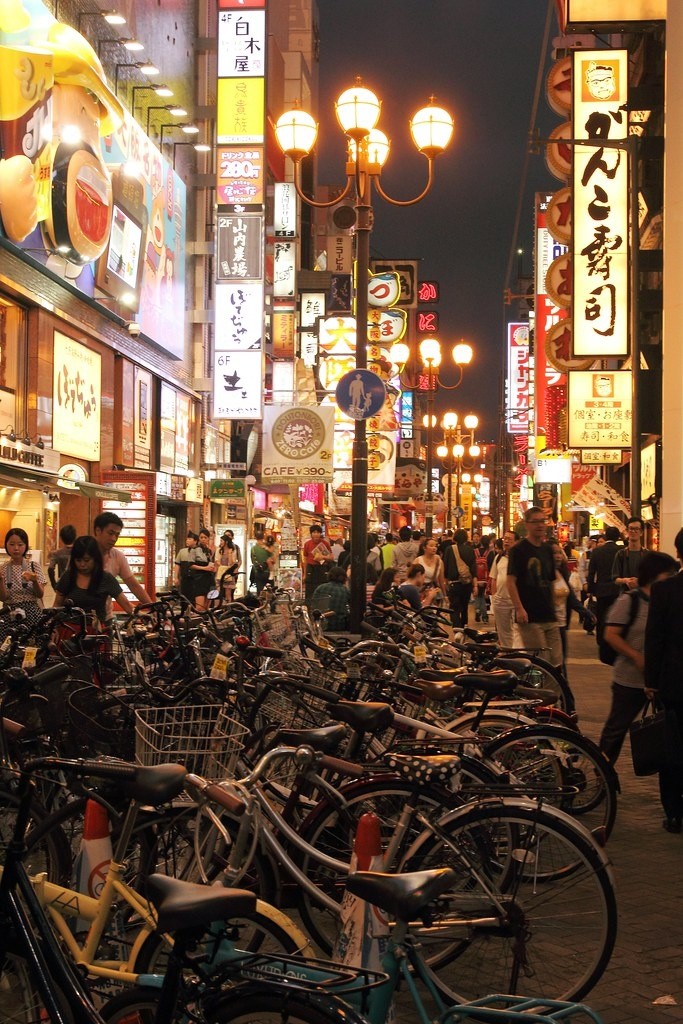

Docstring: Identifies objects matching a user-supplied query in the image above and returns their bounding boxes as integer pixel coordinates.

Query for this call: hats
[188,529,199,541]
[267,535,274,546]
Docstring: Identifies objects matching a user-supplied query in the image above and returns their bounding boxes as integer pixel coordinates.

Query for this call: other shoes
[663,817,683,833]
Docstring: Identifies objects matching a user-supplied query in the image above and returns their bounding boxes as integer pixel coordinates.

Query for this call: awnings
[0,464,132,502]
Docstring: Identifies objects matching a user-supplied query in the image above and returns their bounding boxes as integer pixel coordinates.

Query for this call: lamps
[173,143,211,171]
[160,123,199,154]
[98,37,144,60]
[92,292,136,305]
[78,10,127,34]
[148,105,188,137]
[21,245,72,254]
[132,85,174,118]
[115,62,159,97]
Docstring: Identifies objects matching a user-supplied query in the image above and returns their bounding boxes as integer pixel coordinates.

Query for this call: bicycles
[1,568,626,1024]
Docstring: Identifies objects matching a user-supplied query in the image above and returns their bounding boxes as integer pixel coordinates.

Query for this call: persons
[0,528,47,649]
[92,512,153,605]
[486,531,522,652]
[546,540,598,724]
[303,516,651,633]
[47,524,76,591]
[51,536,149,654]
[398,563,438,612]
[599,550,678,781]
[309,566,352,631]
[371,566,400,615]
[505,506,564,709]
[644,526,683,834]
[174,527,277,615]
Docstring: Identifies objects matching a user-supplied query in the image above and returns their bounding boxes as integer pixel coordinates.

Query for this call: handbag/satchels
[452,544,473,585]
[630,693,670,777]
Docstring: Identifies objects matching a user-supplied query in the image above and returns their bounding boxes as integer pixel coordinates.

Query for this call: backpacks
[473,548,491,583]
[596,590,639,666]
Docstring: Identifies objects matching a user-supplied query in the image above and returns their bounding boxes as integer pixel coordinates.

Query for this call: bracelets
[265,546,269,551]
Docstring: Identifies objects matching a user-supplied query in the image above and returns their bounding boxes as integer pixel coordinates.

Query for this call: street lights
[387,335,473,542]
[274,75,458,648]
[423,409,481,538]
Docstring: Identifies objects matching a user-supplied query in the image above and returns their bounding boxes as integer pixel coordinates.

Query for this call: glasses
[626,527,642,532]
[527,519,549,524]
[502,537,514,542]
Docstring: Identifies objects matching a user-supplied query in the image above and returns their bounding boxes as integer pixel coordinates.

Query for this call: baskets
[135,702,250,807]
[267,615,297,649]
[256,668,383,729]
[97,641,157,689]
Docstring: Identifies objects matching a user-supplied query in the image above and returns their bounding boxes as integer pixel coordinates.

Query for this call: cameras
[10,608,26,621]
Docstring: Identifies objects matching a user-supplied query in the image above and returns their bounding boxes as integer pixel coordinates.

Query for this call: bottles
[209,562,214,569]
[222,553,232,566]
